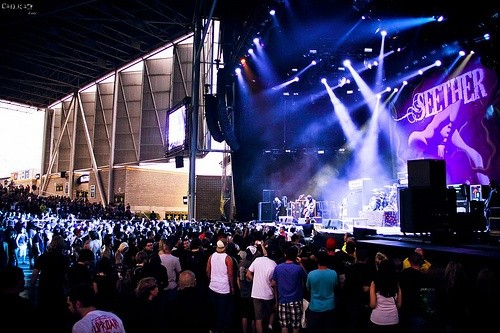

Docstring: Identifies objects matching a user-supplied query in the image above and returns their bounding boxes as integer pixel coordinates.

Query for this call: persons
[0,180,500,333]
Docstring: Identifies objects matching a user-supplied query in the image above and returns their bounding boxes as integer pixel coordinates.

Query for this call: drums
[370,197,388,211]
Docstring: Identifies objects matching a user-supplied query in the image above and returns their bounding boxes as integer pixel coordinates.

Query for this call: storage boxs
[447,184,489,212]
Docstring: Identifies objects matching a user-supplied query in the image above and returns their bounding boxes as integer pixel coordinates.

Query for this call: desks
[359,210,398,227]
[342,218,368,230]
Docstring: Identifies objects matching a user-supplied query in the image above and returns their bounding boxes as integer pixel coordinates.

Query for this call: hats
[216,238,227,252]
[326,237,336,248]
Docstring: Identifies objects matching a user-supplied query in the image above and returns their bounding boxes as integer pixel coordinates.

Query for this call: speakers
[400,159,457,234]
[205,68,240,152]
[258,189,282,222]
